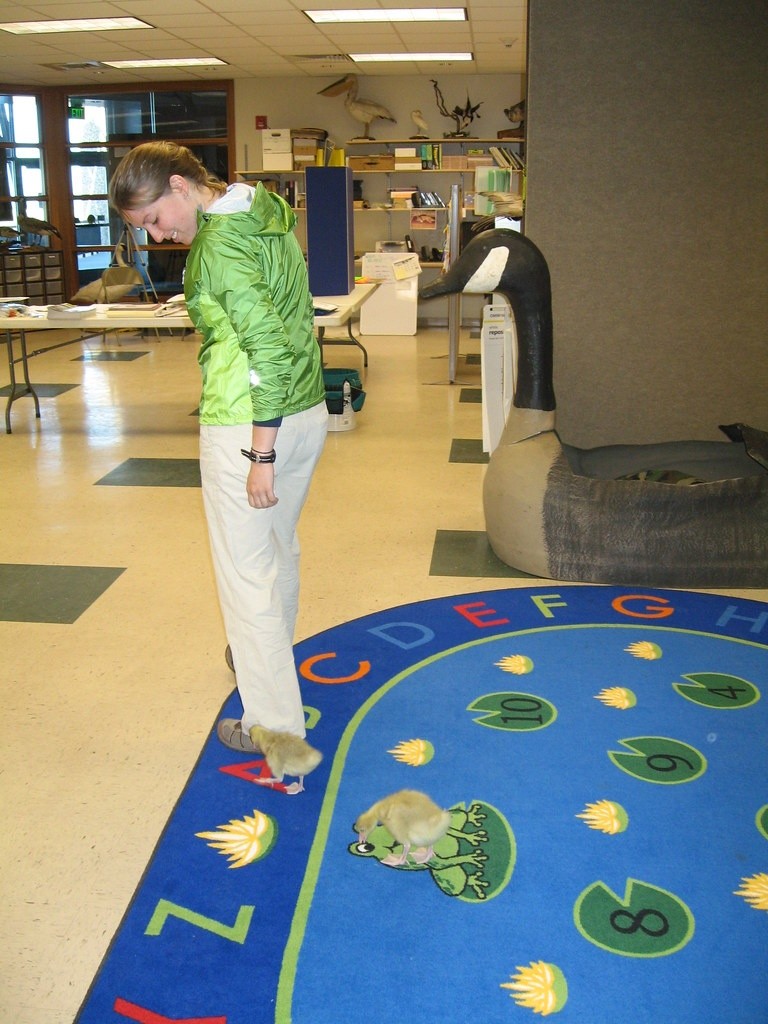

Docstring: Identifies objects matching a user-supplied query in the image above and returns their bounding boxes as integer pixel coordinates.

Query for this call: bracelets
[240,447,277,463]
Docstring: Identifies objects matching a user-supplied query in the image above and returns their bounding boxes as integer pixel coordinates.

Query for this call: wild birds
[69,241,137,304]
[419,229,766,592]
[0,195,63,250]
[504,100,525,128]
[316,73,397,141]
[453,97,484,128]
[411,110,429,136]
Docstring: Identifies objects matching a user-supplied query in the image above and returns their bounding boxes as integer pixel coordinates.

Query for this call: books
[285,180,298,208]
[0,293,190,318]
[351,142,524,212]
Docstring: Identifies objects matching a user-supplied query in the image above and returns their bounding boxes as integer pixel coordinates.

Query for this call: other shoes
[225,644,236,672]
[217,718,262,753]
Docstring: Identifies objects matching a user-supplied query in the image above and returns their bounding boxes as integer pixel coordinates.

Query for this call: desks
[0,283,381,434]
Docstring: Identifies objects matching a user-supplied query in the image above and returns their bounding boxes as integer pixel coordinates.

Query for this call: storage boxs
[394,148,416,157]
[466,154,494,169]
[349,155,395,170]
[442,156,468,169]
[261,128,292,153]
[263,153,293,170]
[395,157,422,170]
[293,138,317,171]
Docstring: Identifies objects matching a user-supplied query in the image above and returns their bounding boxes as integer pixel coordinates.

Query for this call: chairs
[101,267,161,347]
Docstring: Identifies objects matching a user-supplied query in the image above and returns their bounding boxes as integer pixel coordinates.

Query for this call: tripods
[80,221,174,343]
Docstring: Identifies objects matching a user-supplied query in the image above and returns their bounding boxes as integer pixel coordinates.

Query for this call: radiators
[480,304,515,458]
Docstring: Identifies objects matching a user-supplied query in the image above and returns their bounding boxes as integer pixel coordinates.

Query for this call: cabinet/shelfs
[233,170,307,261]
[344,138,527,328]
[0,250,66,306]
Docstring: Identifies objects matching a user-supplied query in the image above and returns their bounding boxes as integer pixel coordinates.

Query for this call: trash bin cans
[322,368,366,432]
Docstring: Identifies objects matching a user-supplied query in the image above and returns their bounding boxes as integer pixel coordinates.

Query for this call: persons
[107,138,329,753]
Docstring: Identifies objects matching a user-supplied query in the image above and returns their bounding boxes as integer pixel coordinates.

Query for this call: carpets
[73,587,768,1024]
